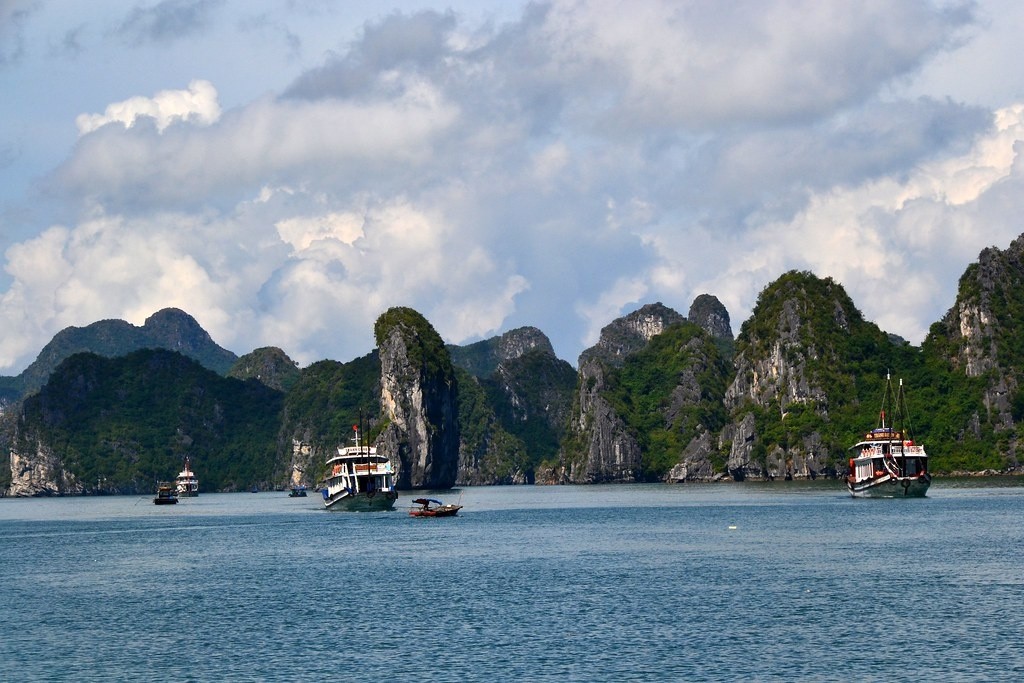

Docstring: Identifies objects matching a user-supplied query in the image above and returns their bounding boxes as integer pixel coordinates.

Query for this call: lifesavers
[367,491,375,498]
[890,473,932,488]
[348,493,354,498]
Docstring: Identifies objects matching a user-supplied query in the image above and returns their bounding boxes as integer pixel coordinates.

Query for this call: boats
[320,409,398,511]
[176,455,199,496]
[844,369,932,498]
[408,491,464,517]
[153,480,179,505]
[251,484,258,493]
[289,485,307,497]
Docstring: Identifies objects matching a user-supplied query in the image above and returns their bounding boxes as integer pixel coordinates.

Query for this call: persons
[424,504,433,512]
